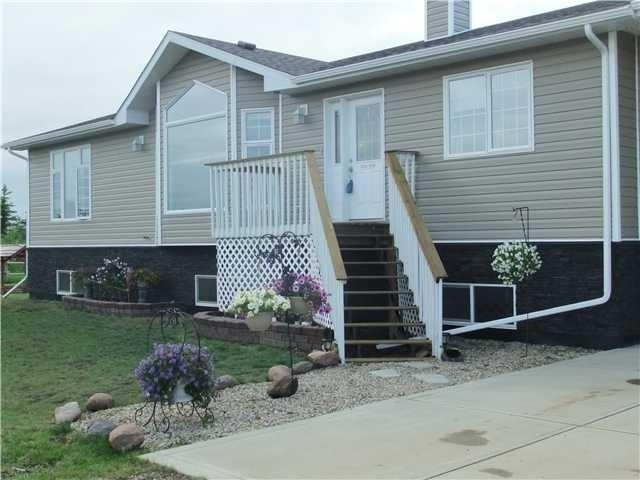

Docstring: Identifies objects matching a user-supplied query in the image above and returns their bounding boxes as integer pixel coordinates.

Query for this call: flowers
[223,271,333,319]
[491,241,542,286]
[91,256,162,289]
[135,342,232,430]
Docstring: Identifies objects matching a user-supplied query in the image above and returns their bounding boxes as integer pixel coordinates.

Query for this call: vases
[91,279,156,304]
[165,376,197,404]
[241,312,274,332]
